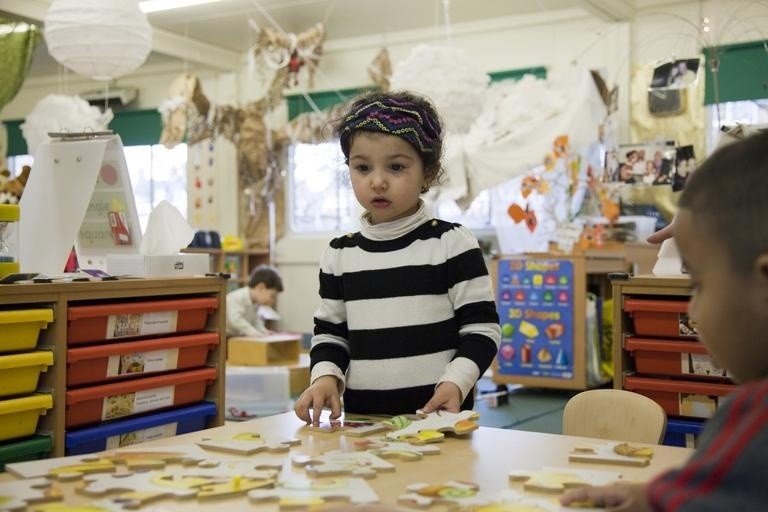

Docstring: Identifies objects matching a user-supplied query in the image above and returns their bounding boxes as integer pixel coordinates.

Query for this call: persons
[223,264,282,340]
[665,58,692,84]
[558,126,767,510]
[292,88,503,430]
[615,149,695,194]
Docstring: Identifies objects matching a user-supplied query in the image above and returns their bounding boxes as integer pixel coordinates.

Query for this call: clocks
[648,89,689,121]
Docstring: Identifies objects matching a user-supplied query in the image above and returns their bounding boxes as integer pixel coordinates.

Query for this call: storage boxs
[1,293,222,465]
[619,294,738,449]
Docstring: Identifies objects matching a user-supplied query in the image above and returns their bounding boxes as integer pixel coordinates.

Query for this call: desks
[0,407,699,511]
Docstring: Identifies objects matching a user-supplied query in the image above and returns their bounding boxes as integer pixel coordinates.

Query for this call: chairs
[562,388,669,446]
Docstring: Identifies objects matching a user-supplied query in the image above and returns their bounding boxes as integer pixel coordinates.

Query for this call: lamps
[39,3,154,80]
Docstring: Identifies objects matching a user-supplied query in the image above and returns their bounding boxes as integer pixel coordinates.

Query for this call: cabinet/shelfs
[489,254,627,396]
[0,270,229,476]
[607,272,739,449]
